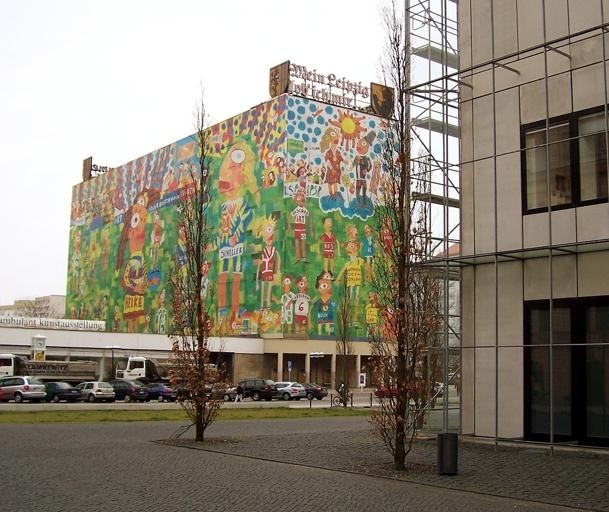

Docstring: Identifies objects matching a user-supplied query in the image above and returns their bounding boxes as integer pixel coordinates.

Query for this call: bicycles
[333,395,353,407]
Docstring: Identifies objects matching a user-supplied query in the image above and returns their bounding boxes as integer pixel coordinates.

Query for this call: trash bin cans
[437,433,458,474]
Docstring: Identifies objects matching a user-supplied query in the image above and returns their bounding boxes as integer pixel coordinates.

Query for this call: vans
[272,382,306,401]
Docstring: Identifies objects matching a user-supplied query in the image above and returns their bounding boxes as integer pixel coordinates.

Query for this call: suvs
[236,378,278,401]
[448,369,460,381]
[111,379,148,403]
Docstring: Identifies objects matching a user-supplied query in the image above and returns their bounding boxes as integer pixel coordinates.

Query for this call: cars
[29,382,81,403]
[145,383,176,403]
[421,381,444,398]
[374,382,398,395]
[75,382,116,403]
[210,382,238,402]
[173,383,212,402]
[295,383,328,400]
[0,374,48,403]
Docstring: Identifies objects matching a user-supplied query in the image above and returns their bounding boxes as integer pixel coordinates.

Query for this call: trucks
[0,353,99,383]
[115,356,219,384]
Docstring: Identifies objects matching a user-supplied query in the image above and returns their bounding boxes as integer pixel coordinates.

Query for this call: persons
[235,384,243,403]
